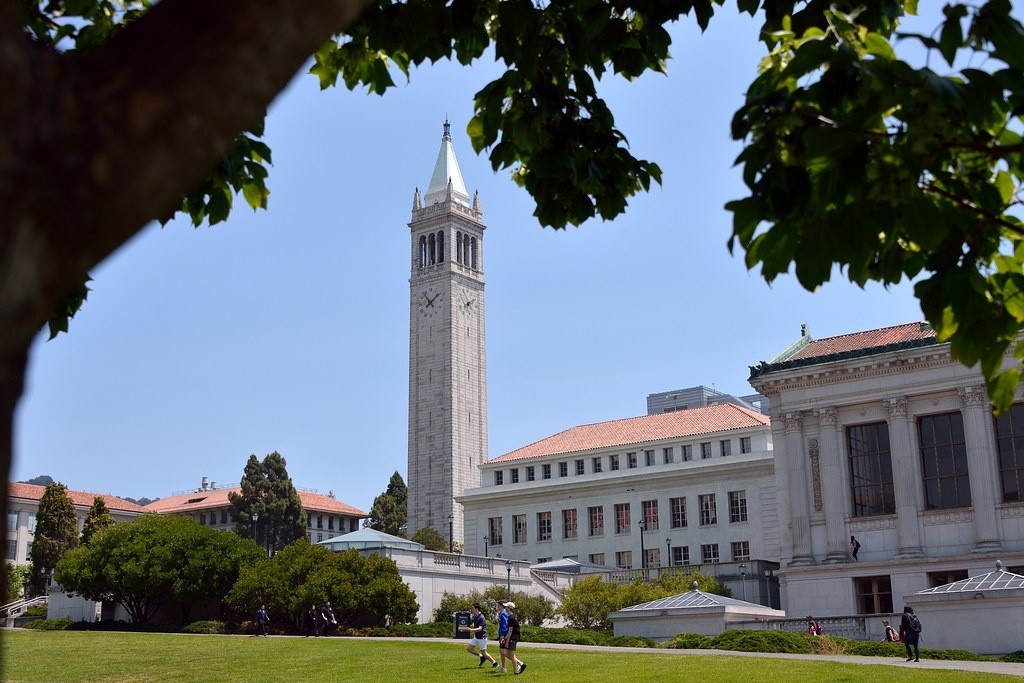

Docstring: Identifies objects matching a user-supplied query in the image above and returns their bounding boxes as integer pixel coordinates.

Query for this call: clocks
[456,288,479,321]
[418,286,445,319]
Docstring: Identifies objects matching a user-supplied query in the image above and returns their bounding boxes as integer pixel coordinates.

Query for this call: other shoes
[907,658,920,662]
[498,664,527,675]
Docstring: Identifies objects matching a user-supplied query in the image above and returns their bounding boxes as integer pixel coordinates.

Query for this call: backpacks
[886,626,899,639]
[907,613,921,632]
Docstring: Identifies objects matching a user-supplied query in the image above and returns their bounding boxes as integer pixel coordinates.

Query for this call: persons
[322,601,335,637]
[253,603,270,636]
[896,607,920,662]
[466,603,500,667]
[305,605,319,637]
[497,600,527,674]
[880,621,895,642]
[851,536,861,562]
[808,616,821,635]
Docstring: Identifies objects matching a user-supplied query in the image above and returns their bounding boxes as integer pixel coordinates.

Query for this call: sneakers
[492,662,500,668]
[479,656,487,666]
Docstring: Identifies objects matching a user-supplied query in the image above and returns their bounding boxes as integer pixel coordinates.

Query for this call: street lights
[638,520,645,578]
[763,568,772,606]
[738,563,748,601]
[666,537,673,567]
[483,536,490,558]
[40,566,47,598]
[252,512,260,545]
[505,560,513,602]
[448,513,455,554]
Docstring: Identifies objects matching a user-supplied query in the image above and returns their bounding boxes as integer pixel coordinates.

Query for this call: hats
[882,620,888,623]
[502,601,516,610]
[497,599,507,605]
